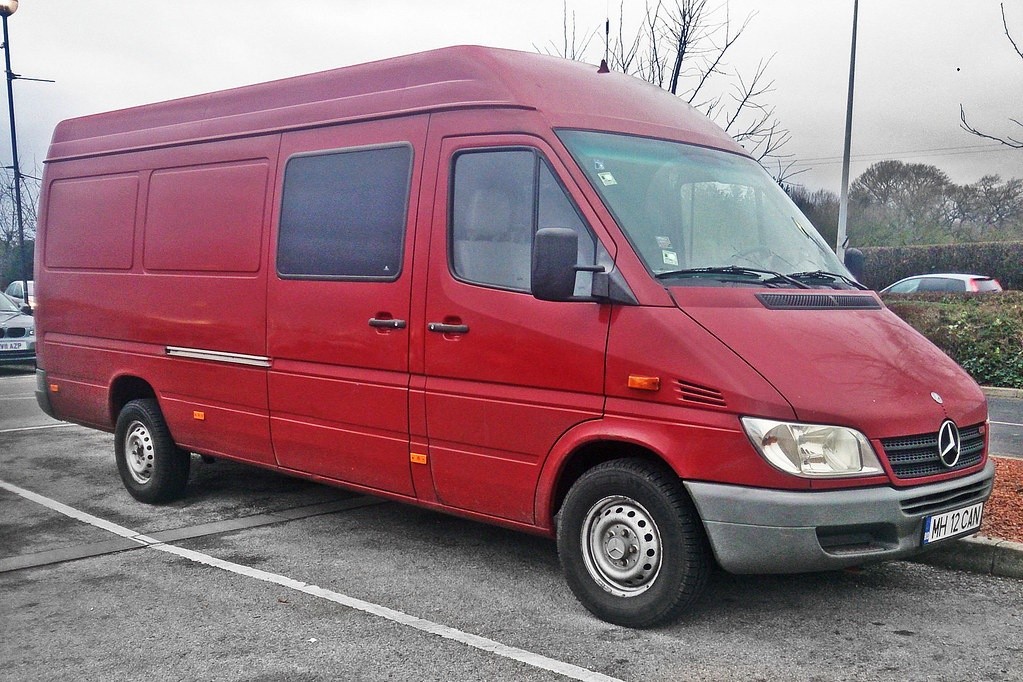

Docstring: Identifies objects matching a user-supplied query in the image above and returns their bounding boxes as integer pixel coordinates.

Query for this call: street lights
[0,0,28,306]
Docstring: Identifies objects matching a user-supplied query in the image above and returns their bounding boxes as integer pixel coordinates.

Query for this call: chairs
[454,189,532,289]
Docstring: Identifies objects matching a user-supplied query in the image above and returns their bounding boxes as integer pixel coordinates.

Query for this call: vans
[35,45,995,630]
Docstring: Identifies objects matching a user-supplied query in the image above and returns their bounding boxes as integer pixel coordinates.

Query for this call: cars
[880,274,1002,292]
[0,280,37,364]
